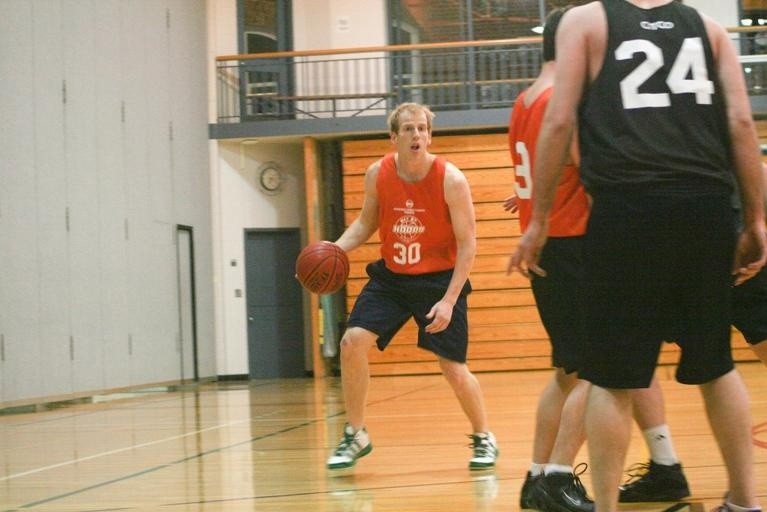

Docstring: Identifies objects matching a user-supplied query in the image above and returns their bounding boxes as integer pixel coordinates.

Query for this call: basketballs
[295,240,350,295]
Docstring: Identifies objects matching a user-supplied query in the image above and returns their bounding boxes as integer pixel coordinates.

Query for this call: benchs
[274,91,398,121]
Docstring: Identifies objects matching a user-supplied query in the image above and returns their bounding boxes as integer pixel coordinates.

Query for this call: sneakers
[467,431,499,470]
[326,421,372,470]
[519,459,762,512]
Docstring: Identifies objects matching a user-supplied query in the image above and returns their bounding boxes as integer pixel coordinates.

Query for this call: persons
[295,103,500,470]
[508,0,766,512]
[503,159,767,501]
[508,5,602,511]
